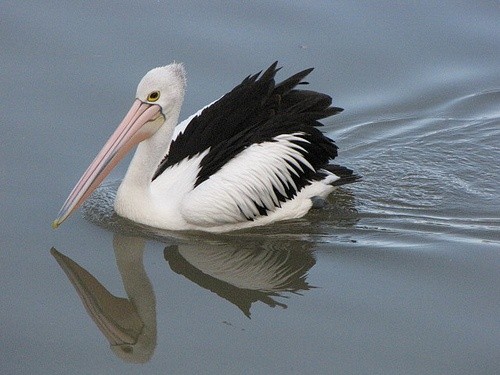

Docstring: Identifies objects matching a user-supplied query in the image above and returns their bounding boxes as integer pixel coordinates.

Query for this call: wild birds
[49,233,326,367]
[51,59,363,234]
